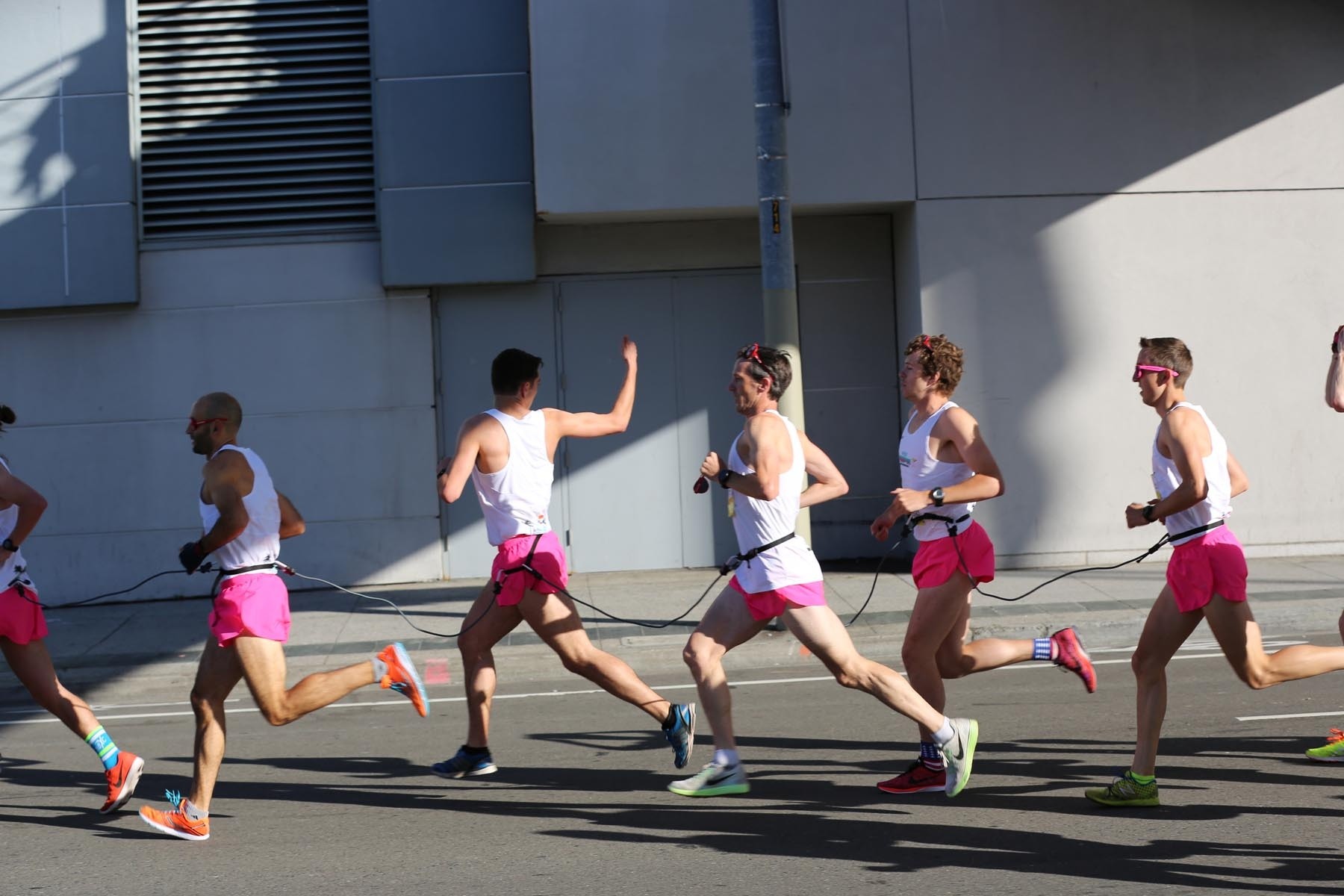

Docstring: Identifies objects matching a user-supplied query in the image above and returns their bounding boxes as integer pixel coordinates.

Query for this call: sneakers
[100,750,145,813]
[666,760,749,797]
[1085,770,1160,806]
[661,703,696,768]
[431,745,497,778]
[1051,624,1096,692]
[1305,728,1344,762]
[876,755,947,794]
[376,641,430,717]
[138,788,210,840]
[933,717,978,798]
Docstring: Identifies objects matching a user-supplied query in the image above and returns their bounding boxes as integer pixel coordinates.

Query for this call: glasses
[920,333,934,353]
[743,343,775,384]
[1134,364,1180,379]
[189,417,228,429]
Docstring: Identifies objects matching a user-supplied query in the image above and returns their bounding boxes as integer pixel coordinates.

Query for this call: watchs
[931,486,945,507]
[1142,505,1158,522]
[717,469,735,489]
[3,538,19,552]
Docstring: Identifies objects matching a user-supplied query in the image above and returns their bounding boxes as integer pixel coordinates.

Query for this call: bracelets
[436,467,446,479]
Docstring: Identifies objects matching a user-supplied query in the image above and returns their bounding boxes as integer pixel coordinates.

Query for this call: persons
[433,332,697,778]
[1085,335,1344,811]
[1305,328,1344,763]
[877,333,1098,796]
[666,342,979,798]
[0,400,144,818]
[138,392,432,843]
